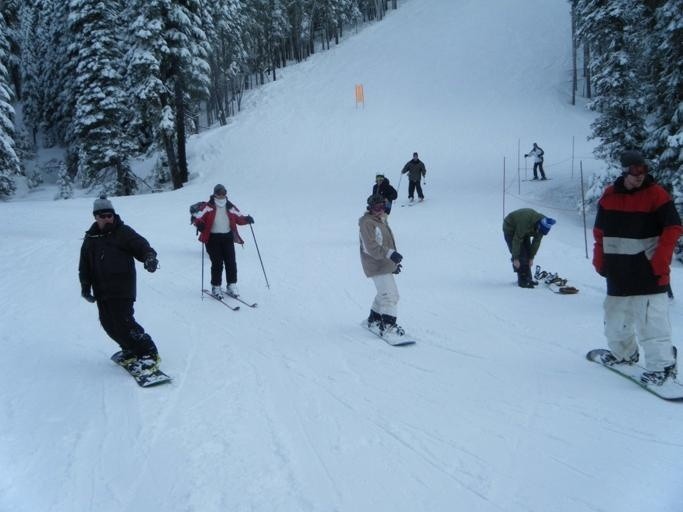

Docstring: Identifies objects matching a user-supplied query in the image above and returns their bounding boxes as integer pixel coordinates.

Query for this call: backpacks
[190,201,208,232]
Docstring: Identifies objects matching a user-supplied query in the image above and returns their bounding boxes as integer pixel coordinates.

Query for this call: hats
[93,194,115,214]
[376,173,385,182]
[214,183,227,193]
[620,151,646,174]
[538,216,557,235]
[367,194,386,205]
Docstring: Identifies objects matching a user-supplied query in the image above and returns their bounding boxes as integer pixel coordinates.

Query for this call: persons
[359,193,405,336]
[79,192,159,375]
[591,149,682,386]
[503,208,556,288]
[373,175,398,216]
[525,143,546,180]
[401,152,426,201]
[189,184,253,299]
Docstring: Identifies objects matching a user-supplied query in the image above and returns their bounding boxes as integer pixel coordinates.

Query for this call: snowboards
[111,350,171,387]
[544,272,579,294]
[586,348,683,400]
[362,319,416,345]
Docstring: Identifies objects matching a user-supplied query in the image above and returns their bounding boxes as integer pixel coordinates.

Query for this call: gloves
[244,215,255,224]
[195,221,207,232]
[390,251,403,262]
[395,263,402,274]
[80,283,98,304]
[144,251,159,273]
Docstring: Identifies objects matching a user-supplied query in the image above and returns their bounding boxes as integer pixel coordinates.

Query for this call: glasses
[622,163,649,176]
[98,212,114,219]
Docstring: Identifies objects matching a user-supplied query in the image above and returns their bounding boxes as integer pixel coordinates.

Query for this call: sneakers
[116,351,137,366]
[601,351,640,365]
[641,368,678,384]
[129,352,163,376]
[226,284,240,297]
[212,285,224,300]
[408,197,414,203]
[418,196,425,202]
[366,317,382,328]
[518,278,539,289]
[379,323,406,336]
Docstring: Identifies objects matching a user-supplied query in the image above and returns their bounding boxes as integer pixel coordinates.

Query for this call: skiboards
[523,178,552,181]
[202,288,257,310]
[401,199,425,207]
